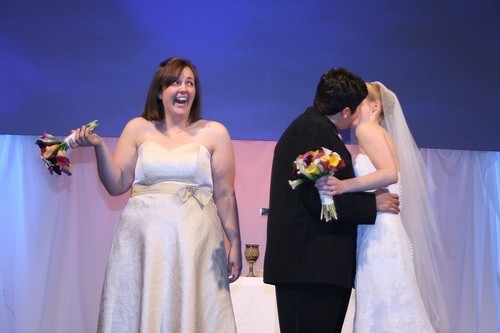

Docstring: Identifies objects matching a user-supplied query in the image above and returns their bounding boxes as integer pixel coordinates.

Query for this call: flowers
[288,146,347,222]
[35,120,99,176]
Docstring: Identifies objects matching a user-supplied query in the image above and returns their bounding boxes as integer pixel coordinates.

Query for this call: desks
[228,275,356,333]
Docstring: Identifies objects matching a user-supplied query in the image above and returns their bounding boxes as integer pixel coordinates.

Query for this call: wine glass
[243,243,260,277]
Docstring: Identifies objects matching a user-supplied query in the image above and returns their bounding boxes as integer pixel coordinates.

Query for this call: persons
[263,67,400,333]
[315,81,437,333]
[68,56,242,333]
